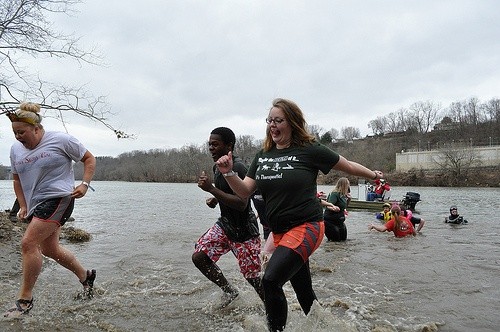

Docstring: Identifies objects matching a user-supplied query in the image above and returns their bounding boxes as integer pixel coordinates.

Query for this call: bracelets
[81,182,95,191]
[222,171,235,177]
[371,172,378,180]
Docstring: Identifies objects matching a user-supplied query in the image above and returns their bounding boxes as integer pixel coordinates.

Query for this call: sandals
[3,297,34,318]
[79,269,97,293]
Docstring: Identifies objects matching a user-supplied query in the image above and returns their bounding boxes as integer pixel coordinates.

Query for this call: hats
[450,207,457,211]
[391,205,401,212]
[382,202,390,208]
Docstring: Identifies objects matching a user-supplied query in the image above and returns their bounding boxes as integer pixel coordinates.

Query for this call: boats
[322,184,422,212]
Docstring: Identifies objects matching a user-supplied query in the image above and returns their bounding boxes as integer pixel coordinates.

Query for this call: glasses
[265,117,287,125]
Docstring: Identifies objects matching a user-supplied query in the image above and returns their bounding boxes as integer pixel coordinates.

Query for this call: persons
[192,127,468,311]
[4,103,97,322]
[217,98,383,332]
